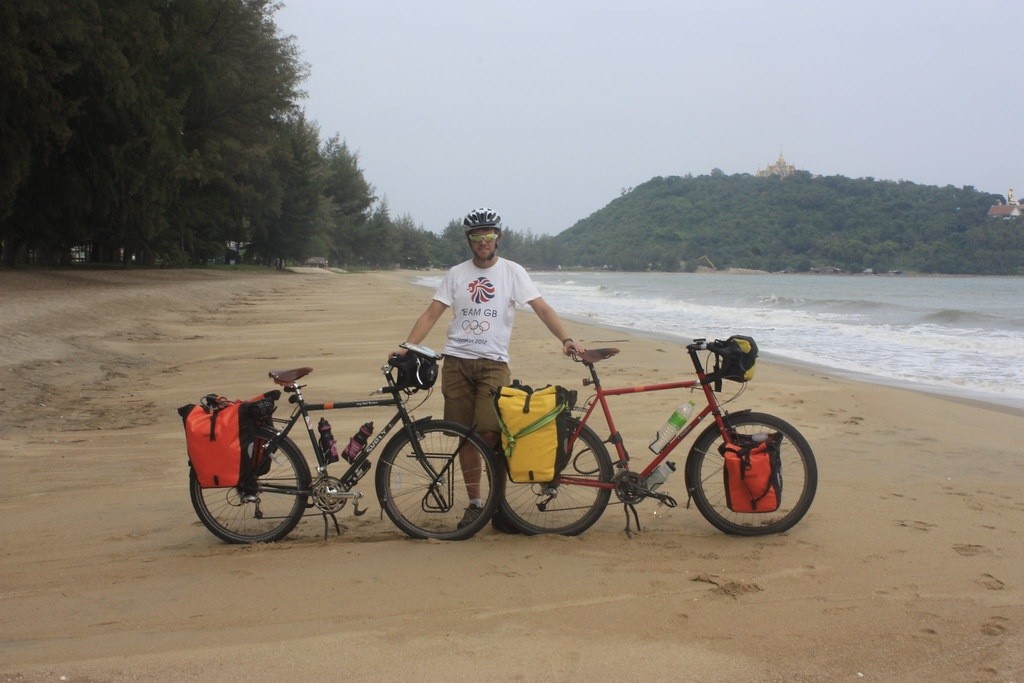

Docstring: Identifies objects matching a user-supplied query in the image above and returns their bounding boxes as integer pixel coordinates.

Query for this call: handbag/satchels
[718,430,783,514]
[493,379,577,483]
[178,390,282,489]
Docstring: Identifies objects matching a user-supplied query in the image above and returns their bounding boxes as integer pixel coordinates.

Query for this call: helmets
[463,207,502,232]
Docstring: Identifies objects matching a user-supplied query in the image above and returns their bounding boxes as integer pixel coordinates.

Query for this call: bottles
[646,461,676,491]
[341,421,374,464]
[317,417,339,463]
[648,400,695,453]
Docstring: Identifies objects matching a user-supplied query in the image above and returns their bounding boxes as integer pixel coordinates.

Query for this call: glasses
[468,232,500,242]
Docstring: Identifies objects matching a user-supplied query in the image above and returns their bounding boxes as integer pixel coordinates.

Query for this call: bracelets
[562,338,575,346]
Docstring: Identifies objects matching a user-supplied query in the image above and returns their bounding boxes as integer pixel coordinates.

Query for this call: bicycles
[187,347,506,546]
[490,335,819,537]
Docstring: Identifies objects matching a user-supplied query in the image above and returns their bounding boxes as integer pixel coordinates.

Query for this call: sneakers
[491,512,520,533]
[457,503,484,529]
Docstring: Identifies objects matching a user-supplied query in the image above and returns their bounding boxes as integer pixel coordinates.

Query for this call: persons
[387,207,588,534]
